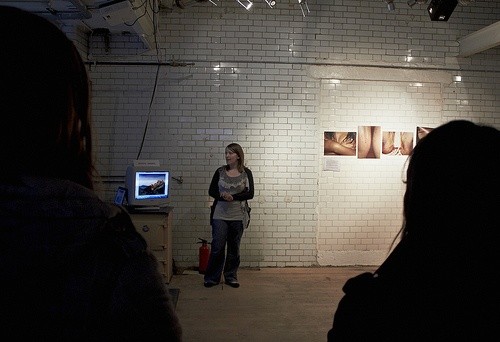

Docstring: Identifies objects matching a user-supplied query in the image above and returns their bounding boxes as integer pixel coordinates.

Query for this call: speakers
[428,0,458,21]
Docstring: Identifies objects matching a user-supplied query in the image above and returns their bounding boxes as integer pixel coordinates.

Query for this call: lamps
[265,0,276,7]
[387,2,395,10]
[407,0,417,8]
[427,0,458,22]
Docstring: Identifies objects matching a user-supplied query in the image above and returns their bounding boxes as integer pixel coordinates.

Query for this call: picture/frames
[113,186,127,204]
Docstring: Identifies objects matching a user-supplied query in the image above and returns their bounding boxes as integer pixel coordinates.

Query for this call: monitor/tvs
[125,166,172,205]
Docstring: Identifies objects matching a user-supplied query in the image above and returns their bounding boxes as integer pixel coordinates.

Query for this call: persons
[202,142,254,289]
[0,5,183,342]
[327,119,499,342]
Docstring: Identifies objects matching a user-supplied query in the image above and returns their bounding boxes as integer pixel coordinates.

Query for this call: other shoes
[204,280,217,287]
[225,280,240,287]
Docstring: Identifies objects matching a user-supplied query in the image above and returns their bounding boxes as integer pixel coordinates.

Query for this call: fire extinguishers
[197,237,210,274]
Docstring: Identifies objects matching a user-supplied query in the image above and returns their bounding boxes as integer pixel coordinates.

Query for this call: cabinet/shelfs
[123,206,175,285]
